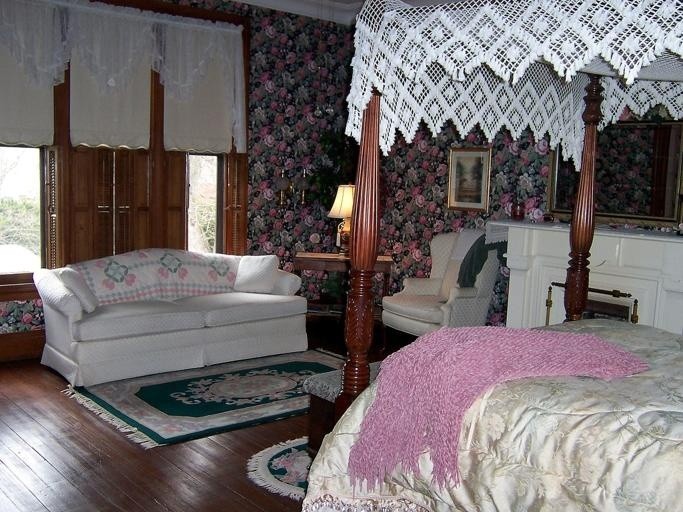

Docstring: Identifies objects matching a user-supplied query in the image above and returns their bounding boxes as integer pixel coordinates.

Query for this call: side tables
[292,252,393,297]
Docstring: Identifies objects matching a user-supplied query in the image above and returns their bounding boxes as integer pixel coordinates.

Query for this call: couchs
[32,247,309,389]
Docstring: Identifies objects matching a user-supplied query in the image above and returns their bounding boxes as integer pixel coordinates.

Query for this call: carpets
[246,436,309,502]
[61,347,347,450]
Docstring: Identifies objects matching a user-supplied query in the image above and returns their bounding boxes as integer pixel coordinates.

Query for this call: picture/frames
[447,147,492,213]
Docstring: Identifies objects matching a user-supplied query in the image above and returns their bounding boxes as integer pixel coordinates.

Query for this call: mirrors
[545,120,683,229]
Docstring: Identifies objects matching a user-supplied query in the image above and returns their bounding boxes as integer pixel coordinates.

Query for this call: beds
[300,0,682,511]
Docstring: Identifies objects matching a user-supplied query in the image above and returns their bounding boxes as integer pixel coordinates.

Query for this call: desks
[485,221,682,334]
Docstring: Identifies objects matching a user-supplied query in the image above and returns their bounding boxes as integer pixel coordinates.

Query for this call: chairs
[381,229,499,337]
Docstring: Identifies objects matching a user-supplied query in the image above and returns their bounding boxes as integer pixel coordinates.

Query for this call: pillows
[233,254,280,294]
[59,267,99,313]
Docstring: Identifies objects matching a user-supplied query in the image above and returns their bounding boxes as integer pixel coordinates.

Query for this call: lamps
[327,185,355,257]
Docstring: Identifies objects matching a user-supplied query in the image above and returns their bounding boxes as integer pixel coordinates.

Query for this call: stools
[303,361,380,451]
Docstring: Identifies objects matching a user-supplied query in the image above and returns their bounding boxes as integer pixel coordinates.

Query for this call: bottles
[511,200,525,220]
